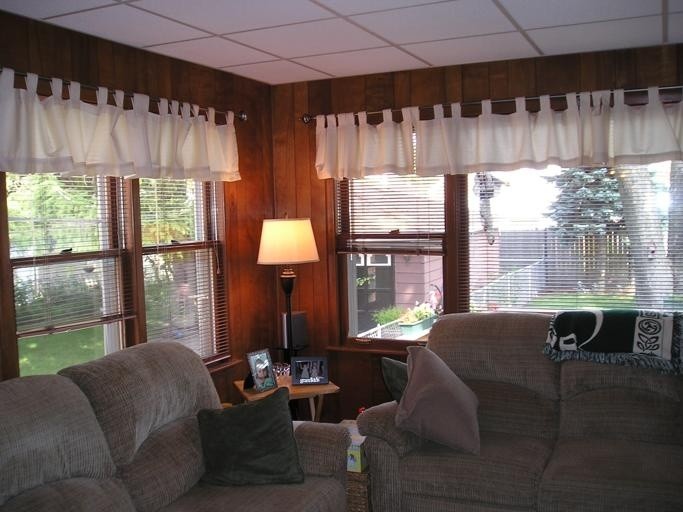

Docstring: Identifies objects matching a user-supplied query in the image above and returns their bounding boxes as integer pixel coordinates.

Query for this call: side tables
[232,375,340,422]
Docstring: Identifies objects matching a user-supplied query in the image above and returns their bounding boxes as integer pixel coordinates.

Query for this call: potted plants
[399,302,436,334]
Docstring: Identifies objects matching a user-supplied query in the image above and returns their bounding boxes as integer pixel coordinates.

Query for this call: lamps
[254,216,321,364]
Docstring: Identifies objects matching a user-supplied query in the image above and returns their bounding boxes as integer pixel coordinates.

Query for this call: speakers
[282,311,307,349]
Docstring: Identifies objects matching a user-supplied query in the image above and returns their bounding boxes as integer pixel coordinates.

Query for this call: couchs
[1,341,353,511]
[356,313,681,510]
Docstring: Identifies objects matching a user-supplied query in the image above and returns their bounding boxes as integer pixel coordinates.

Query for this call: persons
[255,359,274,388]
[301,361,320,379]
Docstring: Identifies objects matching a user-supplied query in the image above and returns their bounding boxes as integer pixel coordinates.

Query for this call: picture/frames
[245,348,278,392]
[291,355,329,386]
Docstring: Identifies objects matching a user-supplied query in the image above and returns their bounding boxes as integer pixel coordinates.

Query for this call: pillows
[378,346,484,452]
[194,384,307,487]
